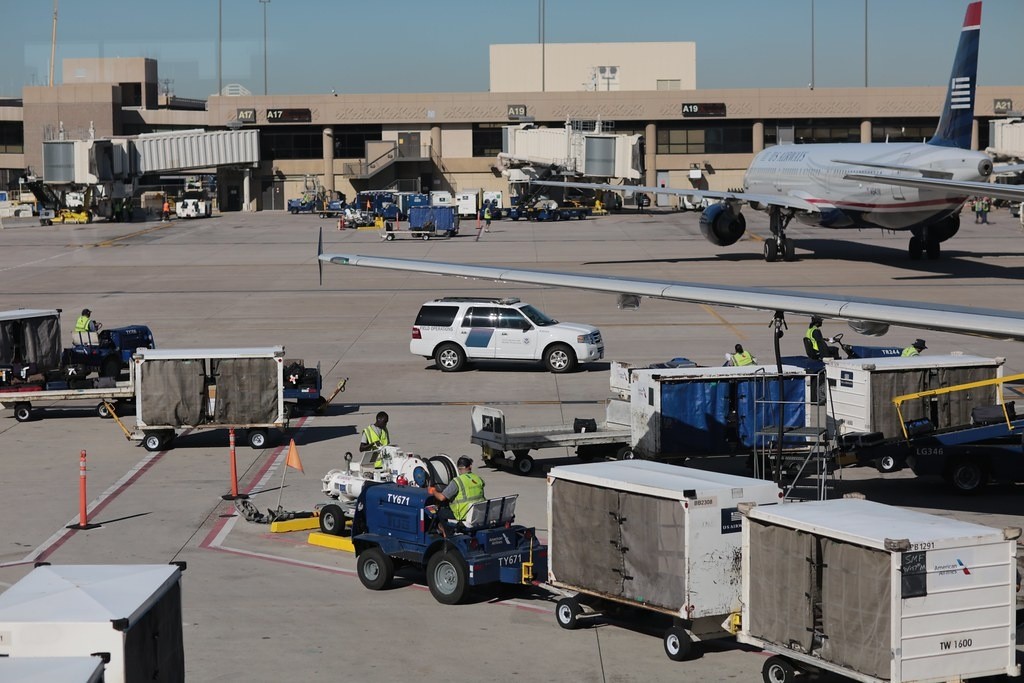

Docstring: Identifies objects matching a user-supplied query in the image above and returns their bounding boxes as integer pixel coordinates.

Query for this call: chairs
[803,337,840,361]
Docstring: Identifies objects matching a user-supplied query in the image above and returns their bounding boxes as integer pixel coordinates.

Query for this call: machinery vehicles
[56,316,162,388]
[352,453,550,604]
[778,330,907,405]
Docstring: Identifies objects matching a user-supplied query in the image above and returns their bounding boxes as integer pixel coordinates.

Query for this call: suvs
[409,296,605,375]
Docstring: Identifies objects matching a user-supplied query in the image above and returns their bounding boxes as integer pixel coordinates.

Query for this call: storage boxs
[629,365,810,457]
[0,560,187,683]
[98,190,213,218]
[826,353,1005,442]
[736,492,1024,683]
[0,308,318,430]
[547,459,783,619]
[330,190,503,230]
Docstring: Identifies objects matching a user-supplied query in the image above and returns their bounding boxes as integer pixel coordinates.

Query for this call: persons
[900,339,928,357]
[615,193,623,210]
[731,344,758,366]
[127,201,134,221]
[806,314,842,360]
[115,200,121,222]
[162,200,171,221]
[636,192,645,211]
[484,203,493,233]
[360,412,396,469]
[969,197,991,224]
[75,308,119,352]
[428,456,488,538]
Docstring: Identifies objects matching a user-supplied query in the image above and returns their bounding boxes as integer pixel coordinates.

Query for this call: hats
[82,309,91,316]
[811,317,823,322]
[457,456,473,468]
[912,339,927,349]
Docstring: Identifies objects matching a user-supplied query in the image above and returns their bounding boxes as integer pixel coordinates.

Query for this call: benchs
[71,331,112,348]
[446,494,519,530]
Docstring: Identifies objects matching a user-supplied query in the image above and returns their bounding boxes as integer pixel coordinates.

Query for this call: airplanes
[506,2,1024,265]
